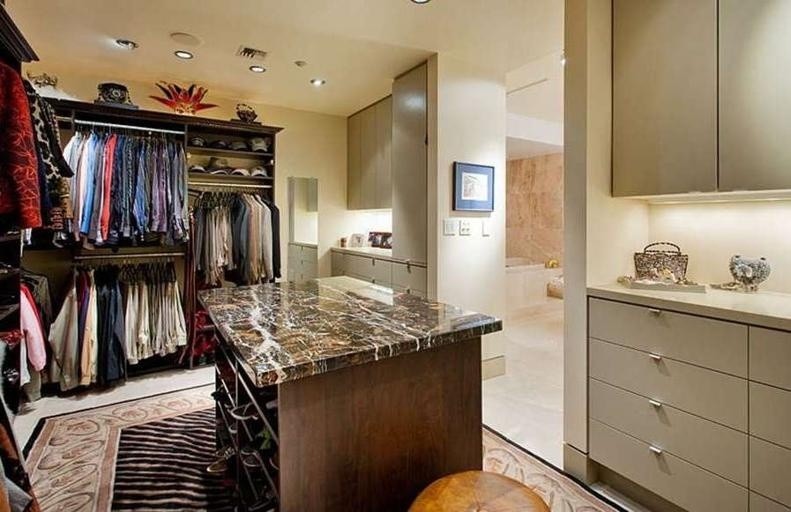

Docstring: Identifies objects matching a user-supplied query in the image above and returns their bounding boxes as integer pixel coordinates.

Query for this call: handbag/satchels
[633,242,689,283]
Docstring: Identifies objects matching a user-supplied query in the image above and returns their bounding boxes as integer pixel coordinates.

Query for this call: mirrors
[287,177,320,277]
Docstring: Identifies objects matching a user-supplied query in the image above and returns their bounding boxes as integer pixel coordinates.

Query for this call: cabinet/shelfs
[197,275,502,510]
[186,116,285,370]
[329,61,429,293]
[23,94,185,395]
[0,6,39,419]
[612,2,791,207]
[588,280,791,510]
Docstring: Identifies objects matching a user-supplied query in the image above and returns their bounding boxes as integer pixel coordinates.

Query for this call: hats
[190,134,270,151]
[188,158,267,177]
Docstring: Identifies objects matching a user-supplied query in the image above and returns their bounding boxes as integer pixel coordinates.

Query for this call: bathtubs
[505,255,547,272]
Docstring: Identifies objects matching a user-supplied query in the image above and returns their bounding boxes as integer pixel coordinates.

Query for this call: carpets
[20,377,633,511]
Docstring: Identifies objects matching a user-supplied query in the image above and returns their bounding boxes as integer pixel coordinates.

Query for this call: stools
[410,471,551,512]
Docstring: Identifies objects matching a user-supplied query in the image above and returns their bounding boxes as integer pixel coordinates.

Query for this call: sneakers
[206,366,278,511]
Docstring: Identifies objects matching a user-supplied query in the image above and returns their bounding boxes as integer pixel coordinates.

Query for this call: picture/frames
[453,160,496,213]
